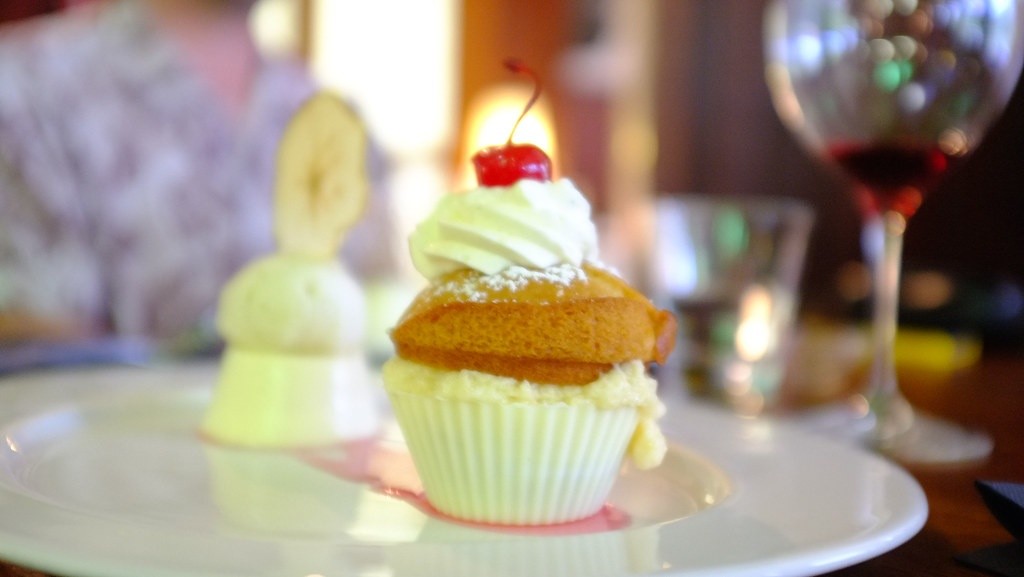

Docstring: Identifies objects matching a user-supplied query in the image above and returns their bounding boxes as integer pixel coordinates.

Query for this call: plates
[0,370,930,577]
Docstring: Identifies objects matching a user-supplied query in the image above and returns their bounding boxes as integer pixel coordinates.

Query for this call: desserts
[201,96,382,448]
[379,58,673,525]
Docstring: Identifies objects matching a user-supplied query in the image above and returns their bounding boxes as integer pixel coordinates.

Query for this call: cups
[640,195,813,407]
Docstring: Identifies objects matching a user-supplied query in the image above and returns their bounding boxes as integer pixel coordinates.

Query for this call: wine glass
[761,0,1024,466]
